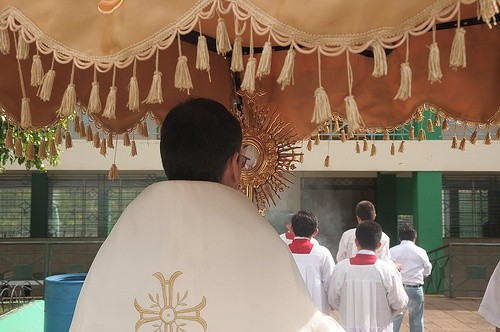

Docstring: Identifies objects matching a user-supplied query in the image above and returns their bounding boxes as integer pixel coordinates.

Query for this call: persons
[328,220,408,332]
[478,261,500,332]
[279,214,320,247]
[282,209,334,315]
[69,98,346,332]
[336,201,390,262]
[386,227,432,332]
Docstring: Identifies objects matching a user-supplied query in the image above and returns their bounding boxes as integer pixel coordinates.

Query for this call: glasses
[236,155,250,168]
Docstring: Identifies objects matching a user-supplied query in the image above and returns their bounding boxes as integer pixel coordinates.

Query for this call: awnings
[1,0,499,149]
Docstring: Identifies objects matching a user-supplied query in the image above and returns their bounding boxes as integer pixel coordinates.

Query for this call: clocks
[226,96,304,219]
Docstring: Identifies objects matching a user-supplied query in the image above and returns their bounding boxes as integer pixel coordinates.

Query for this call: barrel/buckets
[43,273,87,332]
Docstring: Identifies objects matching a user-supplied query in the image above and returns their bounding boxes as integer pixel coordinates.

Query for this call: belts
[402,283,425,289]
